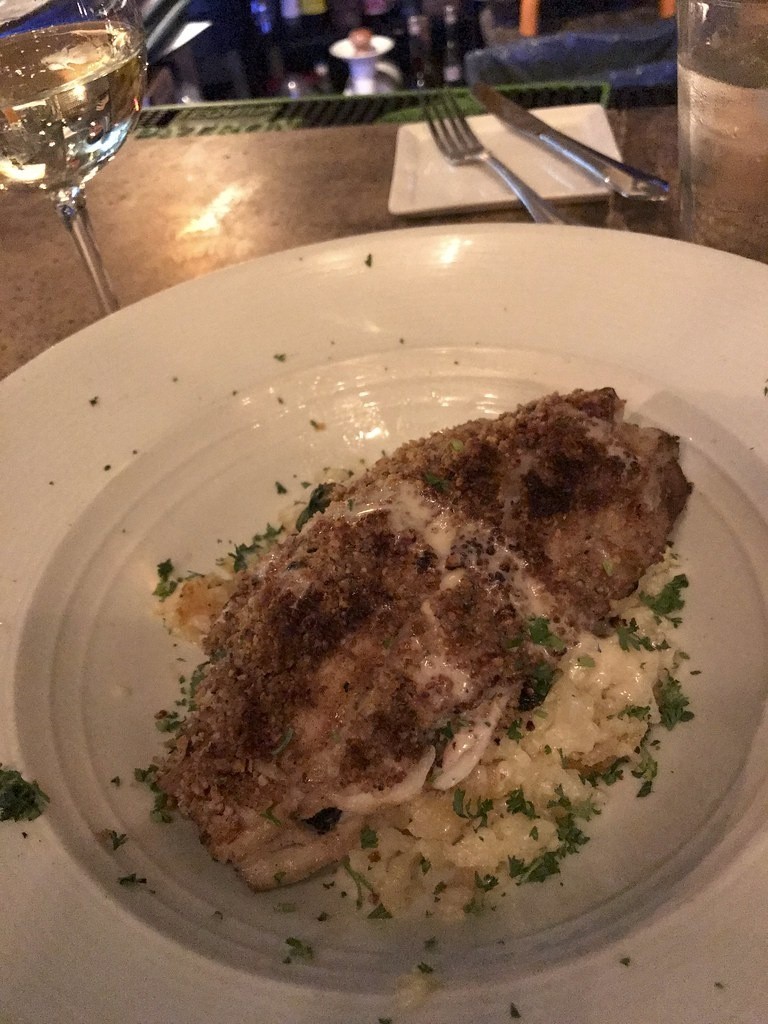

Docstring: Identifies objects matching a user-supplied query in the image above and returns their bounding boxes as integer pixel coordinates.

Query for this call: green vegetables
[105,480,695,974]
[0,763,50,823]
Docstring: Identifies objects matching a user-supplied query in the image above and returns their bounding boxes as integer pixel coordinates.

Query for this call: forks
[420,83,577,227]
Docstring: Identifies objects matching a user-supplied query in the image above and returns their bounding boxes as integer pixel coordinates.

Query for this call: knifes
[469,82,674,206]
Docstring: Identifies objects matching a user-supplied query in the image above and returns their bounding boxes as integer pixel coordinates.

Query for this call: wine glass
[0,0,150,324]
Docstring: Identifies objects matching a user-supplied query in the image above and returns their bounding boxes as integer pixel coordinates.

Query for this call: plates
[327,35,395,60]
[1,220,768,1023]
[388,104,625,217]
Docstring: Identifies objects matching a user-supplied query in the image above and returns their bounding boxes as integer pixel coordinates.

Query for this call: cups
[673,1,768,262]
